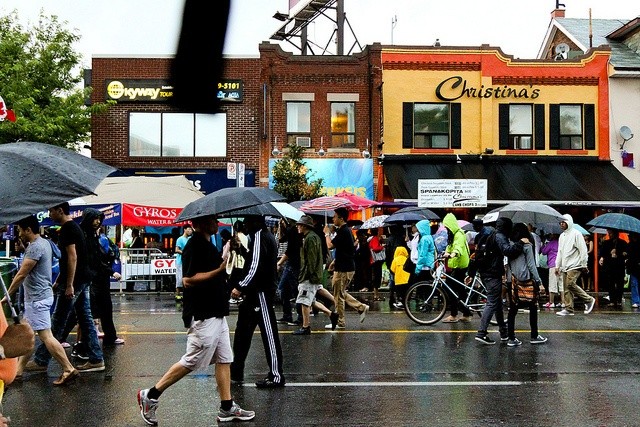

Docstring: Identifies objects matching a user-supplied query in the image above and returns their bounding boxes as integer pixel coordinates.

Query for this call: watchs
[0,344,6,359]
[325,231,332,237]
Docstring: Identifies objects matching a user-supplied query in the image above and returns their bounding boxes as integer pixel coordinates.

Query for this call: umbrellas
[352,214,397,229]
[382,206,442,236]
[173,185,287,222]
[298,195,356,225]
[587,226,611,237]
[218,202,288,221]
[0,142,117,323]
[586,212,640,247]
[543,223,590,234]
[483,201,568,225]
[334,189,386,220]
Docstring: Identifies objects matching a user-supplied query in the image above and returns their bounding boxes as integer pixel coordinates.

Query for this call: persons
[229,215,286,387]
[365,227,386,300]
[220,229,232,260]
[137,214,255,425]
[390,246,410,308]
[228,220,249,303]
[555,213,596,316]
[475,217,531,344]
[348,229,374,304]
[147,234,164,293]
[323,207,370,328]
[0,300,35,403]
[393,221,420,309]
[0,214,81,385]
[414,219,435,310]
[99,232,122,281]
[626,232,640,307]
[123,227,143,292]
[599,227,630,306]
[457,219,475,230]
[443,212,473,323]
[580,235,593,292]
[175,224,195,300]
[80,208,124,343]
[292,215,339,335]
[276,218,302,325]
[541,233,565,307]
[507,223,548,346]
[526,223,543,311]
[386,225,405,309]
[25,201,105,372]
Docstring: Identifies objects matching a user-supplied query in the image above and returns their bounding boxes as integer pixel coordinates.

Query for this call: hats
[296,215,315,228]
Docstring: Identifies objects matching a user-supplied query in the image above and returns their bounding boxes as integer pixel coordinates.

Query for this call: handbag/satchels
[508,259,539,308]
[403,234,419,273]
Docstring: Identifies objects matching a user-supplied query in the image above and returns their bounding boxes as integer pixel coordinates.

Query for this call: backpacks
[475,230,501,273]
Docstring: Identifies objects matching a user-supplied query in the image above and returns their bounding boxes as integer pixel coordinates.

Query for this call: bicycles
[405,254,519,327]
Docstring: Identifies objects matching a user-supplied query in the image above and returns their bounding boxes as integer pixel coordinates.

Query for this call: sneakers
[288,320,303,325]
[359,288,368,292]
[475,335,496,345]
[76,360,105,373]
[556,303,561,306]
[603,296,610,302]
[393,302,405,309]
[556,309,575,316]
[292,326,311,335]
[359,304,369,323]
[584,297,595,314]
[608,303,616,306]
[632,304,638,307]
[53,369,80,385]
[276,318,292,324]
[175,295,183,300]
[501,336,510,341]
[330,312,339,331]
[373,296,385,301]
[61,342,70,348]
[228,297,243,303]
[24,361,48,373]
[542,302,555,308]
[325,323,345,329]
[530,335,548,344]
[113,338,124,344]
[507,338,523,346]
[441,316,458,322]
[137,388,159,425]
[561,302,565,307]
[71,341,90,360]
[216,401,256,422]
[255,377,284,387]
[230,378,244,387]
[458,317,471,322]
